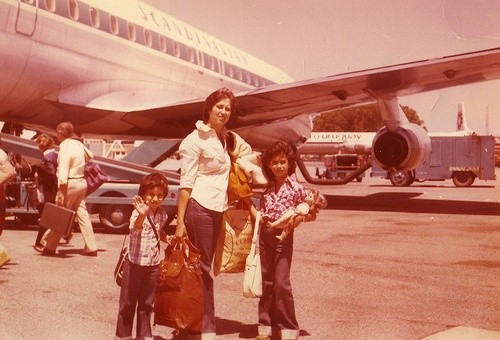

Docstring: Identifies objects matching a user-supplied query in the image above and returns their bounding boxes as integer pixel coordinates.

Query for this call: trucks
[370,133,497,186]
[315,154,369,182]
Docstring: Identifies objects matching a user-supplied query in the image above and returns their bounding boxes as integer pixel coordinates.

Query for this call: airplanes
[299,101,474,153]
[0,1,500,232]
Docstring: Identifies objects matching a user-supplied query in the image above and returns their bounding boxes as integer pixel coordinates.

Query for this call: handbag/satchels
[226,143,253,204]
[153,238,204,333]
[156,237,185,291]
[0,149,17,184]
[243,211,262,298]
[84,148,108,197]
[114,246,128,287]
[214,197,254,277]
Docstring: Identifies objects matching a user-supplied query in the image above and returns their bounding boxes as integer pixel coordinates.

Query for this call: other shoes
[255,335,273,340]
[0,244,11,266]
[34,245,55,256]
[79,249,97,256]
[66,232,75,244]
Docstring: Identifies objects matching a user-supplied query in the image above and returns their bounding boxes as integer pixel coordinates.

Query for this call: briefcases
[39,202,77,240]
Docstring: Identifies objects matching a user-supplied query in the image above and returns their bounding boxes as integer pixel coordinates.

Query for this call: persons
[115,173,176,340]
[256,140,316,340]
[175,88,271,340]
[268,189,327,241]
[0,122,99,269]
